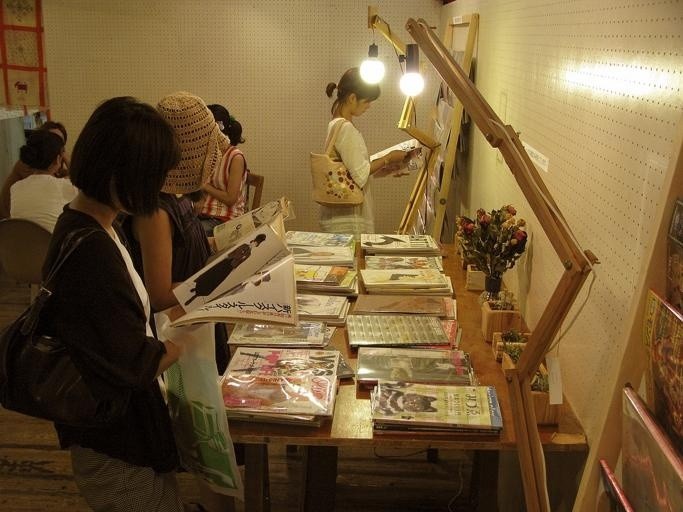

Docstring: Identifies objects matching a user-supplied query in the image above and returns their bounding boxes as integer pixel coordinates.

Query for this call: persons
[8,129,78,235]
[192,105,247,237]
[0,122,67,221]
[130,92,245,468]
[319,66,408,236]
[41,96,183,512]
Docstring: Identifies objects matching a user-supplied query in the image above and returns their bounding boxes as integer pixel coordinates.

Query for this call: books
[370,139,423,180]
[405,42,476,239]
[221,231,504,438]
[171,194,299,329]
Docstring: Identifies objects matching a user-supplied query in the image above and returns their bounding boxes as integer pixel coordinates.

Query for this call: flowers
[454,205,528,277]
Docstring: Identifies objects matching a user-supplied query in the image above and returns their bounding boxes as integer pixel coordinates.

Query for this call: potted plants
[492,328,534,361]
[480,289,520,342]
[529,363,563,426]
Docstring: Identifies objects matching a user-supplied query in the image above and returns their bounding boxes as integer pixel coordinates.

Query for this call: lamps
[400,42,425,98]
[359,44,385,84]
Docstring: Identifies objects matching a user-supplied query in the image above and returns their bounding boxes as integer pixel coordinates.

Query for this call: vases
[478,277,503,307]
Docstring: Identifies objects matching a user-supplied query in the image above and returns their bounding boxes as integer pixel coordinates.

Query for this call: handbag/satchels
[153,311,245,502]
[309,152,364,207]
[0,287,131,428]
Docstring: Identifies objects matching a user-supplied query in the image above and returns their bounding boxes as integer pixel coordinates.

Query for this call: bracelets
[384,157,390,166]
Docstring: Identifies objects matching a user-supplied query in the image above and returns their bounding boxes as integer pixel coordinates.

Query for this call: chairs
[1,217,53,309]
[247,173,265,210]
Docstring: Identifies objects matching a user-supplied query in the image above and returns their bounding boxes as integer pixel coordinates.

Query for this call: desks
[222,245,591,511]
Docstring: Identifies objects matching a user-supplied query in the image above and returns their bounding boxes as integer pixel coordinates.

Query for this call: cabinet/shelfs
[569,140,682,512]
[416,14,480,243]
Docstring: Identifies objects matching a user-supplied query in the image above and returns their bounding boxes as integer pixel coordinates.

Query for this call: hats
[156,91,230,195]
